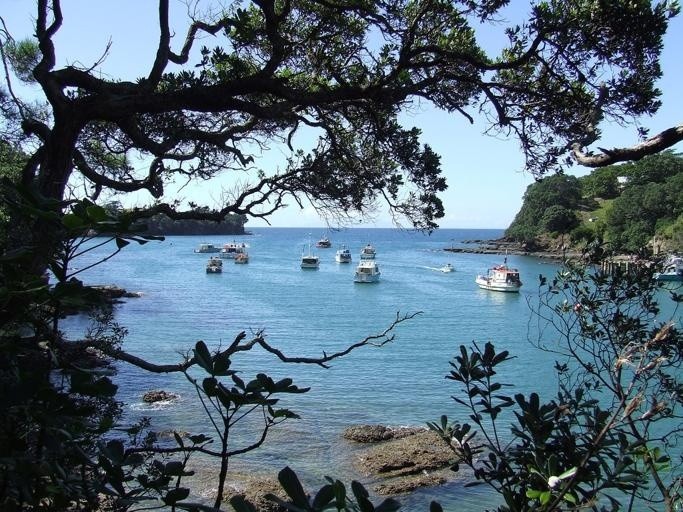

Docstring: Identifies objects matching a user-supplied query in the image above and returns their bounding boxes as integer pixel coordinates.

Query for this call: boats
[354,260,381,283]
[473,250,523,293]
[360,244,377,260]
[477,287,520,306]
[301,232,320,268]
[316,232,331,248]
[441,263,455,274]
[653,259,683,280]
[192,239,249,275]
[335,244,352,264]
